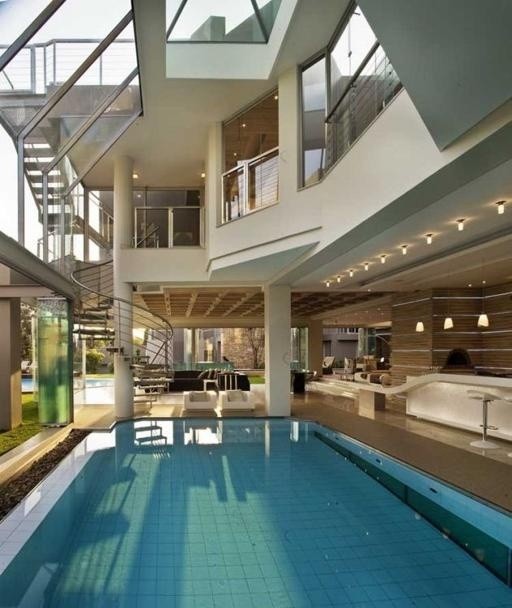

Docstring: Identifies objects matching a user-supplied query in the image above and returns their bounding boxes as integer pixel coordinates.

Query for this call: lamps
[413,255,489,334]
[322,198,508,291]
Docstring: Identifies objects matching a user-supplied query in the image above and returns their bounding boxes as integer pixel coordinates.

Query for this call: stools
[466,388,512,458]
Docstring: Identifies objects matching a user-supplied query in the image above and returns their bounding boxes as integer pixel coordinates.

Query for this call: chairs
[183,388,257,415]
